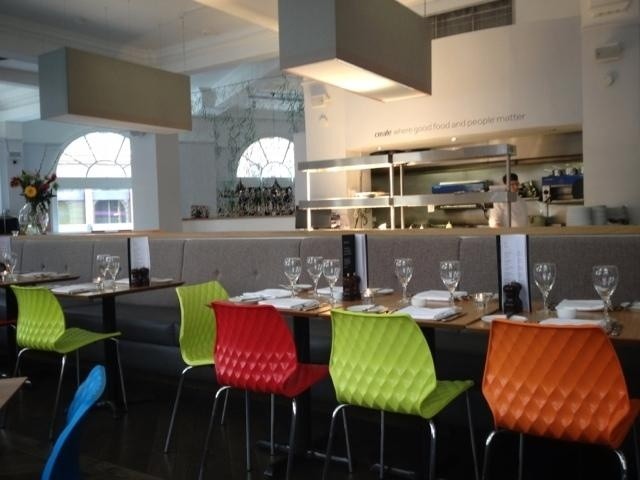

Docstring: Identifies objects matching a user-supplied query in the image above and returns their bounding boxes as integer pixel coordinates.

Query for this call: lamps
[38,0,192,134]
[278,0,432,103]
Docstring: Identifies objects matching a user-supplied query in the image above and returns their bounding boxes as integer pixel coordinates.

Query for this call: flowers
[10,168,57,237]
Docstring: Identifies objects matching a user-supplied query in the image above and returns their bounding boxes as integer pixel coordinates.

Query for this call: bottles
[343,272,361,300]
[131,267,149,287]
[503,281,523,314]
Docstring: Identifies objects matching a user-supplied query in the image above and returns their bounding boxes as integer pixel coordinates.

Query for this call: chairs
[9,284,129,439]
[40,364,107,480]
[163,279,640,479]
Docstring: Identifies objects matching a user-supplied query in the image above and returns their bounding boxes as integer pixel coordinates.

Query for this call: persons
[488,173,529,228]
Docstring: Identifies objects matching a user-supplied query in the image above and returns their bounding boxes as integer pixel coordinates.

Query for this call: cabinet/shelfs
[298,143,517,229]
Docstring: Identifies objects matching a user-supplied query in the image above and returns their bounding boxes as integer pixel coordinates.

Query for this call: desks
[0,375,28,408]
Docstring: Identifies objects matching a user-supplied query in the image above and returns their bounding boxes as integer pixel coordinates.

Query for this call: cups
[411,298,427,307]
[557,307,577,319]
[473,292,487,311]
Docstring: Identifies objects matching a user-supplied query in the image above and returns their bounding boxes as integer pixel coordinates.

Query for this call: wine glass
[4,252,17,278]
[283,255,341,303]
[97,255,120,287]
[392,257,414,305]
[533,263,558,314]
[439,260,462,311]
[592,265,619,327]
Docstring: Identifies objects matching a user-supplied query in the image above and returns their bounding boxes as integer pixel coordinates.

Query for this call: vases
[18,201,49,237]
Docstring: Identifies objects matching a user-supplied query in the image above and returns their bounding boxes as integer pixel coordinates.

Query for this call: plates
[260,288,290,299]
[347,304,388,313]
[567,204,607,227]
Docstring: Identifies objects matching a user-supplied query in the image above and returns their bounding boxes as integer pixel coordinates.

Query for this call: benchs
[0,233,185,348]
[180,223,640,461]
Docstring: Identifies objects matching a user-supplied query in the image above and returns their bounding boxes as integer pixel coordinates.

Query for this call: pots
[544,168,578,176]
